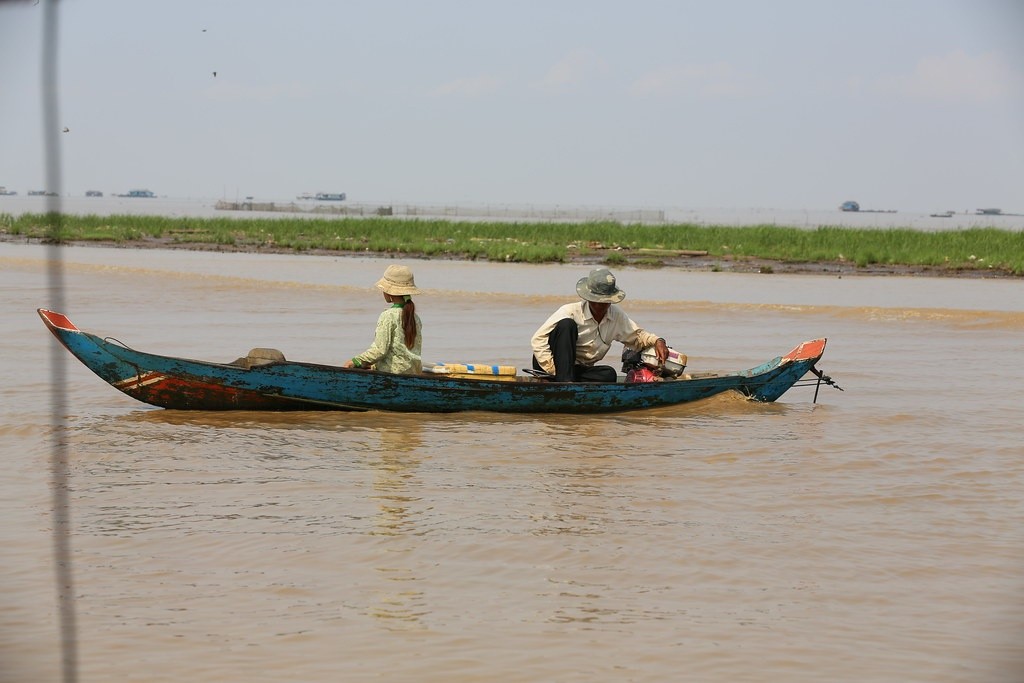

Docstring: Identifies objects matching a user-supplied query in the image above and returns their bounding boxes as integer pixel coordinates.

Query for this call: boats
[37,306,829,418]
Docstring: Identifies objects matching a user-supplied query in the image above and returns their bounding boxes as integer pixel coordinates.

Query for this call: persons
[531,267,670,382]
[343,265,423,376]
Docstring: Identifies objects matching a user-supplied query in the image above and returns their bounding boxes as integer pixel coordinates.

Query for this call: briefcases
[621,344,687,380]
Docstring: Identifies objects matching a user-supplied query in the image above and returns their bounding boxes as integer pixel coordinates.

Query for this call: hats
[576,268,626,304]
[375,265,421,295]
